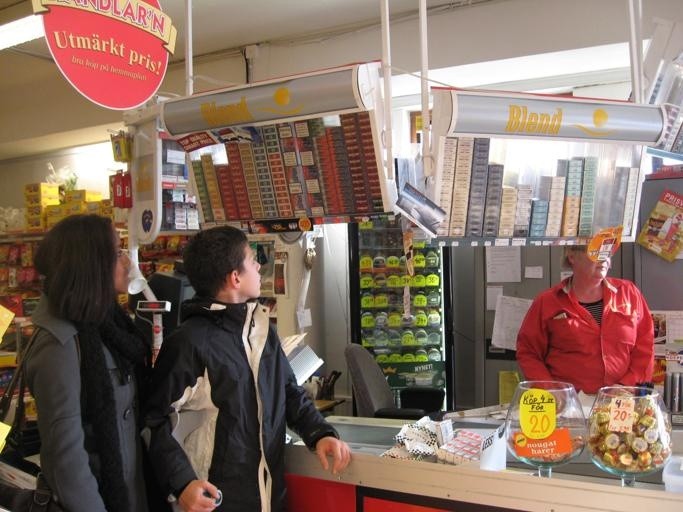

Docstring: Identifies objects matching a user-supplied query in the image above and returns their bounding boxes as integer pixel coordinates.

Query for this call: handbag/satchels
[0,406,56,511]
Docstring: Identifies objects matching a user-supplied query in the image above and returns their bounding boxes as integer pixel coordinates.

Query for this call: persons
[23,214,153,512]
[516,246,655,415]
[138,226,351,512]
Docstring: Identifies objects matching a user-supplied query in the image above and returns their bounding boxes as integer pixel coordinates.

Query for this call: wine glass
[504,379,589,477]
[586,385,673,488]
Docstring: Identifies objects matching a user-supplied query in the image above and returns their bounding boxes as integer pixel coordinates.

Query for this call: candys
[587,390,673,475]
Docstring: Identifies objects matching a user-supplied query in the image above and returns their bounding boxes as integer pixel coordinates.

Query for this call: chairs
[345,343,453,421]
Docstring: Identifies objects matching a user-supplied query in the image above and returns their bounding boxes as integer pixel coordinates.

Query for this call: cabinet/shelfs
[0,227,325,422]
